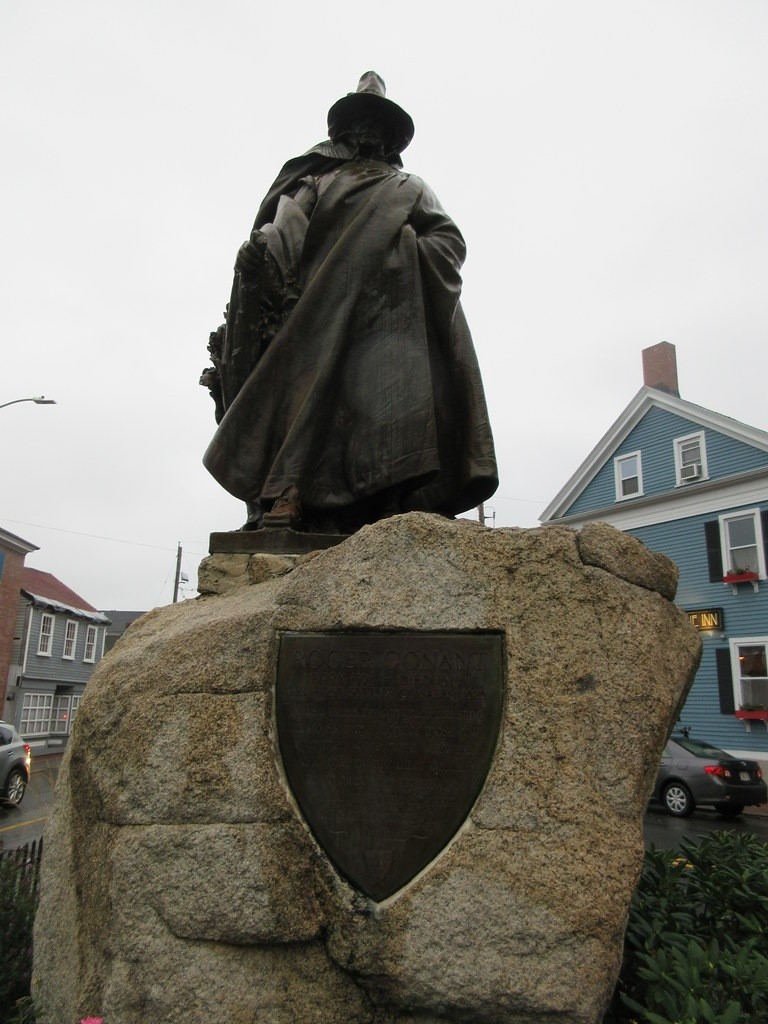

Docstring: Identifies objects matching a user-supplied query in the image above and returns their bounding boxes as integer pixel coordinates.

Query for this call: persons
[201,72,501,538]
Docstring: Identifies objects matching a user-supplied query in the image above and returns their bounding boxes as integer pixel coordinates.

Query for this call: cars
[648,737,768,818]
[1,722,30,809]
